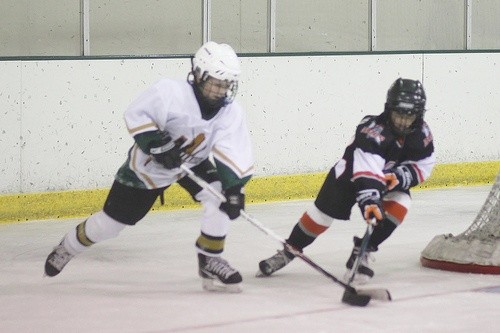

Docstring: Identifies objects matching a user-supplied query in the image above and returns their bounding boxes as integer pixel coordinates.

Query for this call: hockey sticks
[342,191,385,307]
[180,163,392,301]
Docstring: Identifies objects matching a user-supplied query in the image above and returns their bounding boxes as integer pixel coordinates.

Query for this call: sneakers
[343,245,374,284]
[255,249,291,279]
[43,233,75,279]
[197,252,243,294]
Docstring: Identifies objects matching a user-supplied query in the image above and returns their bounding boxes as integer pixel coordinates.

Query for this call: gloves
[382,169,403,194]
[218,184,245,219]
[146,131,182,171]
[357,193,384,226]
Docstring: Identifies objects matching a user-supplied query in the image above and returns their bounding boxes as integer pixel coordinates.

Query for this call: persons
[258,79,436,278]
[45,41,255,285]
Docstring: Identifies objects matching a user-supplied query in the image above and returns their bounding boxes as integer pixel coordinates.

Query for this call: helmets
[190,41,241,82]
[383,77,426,137]
